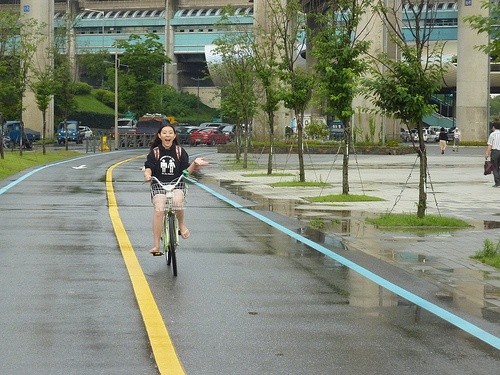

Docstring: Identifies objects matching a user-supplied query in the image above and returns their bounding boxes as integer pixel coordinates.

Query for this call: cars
[400,125,452,143]
[183,123,247,146]
[79,126,93,140]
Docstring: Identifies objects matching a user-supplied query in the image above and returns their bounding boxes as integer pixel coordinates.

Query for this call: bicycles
[142,169,198,276]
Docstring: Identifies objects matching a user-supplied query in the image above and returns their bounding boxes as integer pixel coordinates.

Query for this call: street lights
[161,63,177,105]
[191,77,205,108]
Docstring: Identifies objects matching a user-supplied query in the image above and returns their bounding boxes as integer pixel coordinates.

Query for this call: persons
[439,127,448,154]
[452,128,461,152]
[143,125,208,254]
[485,122,500,188]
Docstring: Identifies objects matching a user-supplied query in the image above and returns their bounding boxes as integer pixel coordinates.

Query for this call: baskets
[150,182,188,211]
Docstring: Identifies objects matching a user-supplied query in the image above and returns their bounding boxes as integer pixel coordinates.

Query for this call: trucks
[110,113,173,147]
[56,121,85,144]
[0,120,41,148]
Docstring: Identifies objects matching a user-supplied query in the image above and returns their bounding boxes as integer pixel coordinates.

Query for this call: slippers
[180,227,191,239]
[150,247,159,253]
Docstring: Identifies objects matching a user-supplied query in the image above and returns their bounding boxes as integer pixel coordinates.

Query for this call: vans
[327,121,345,137]
